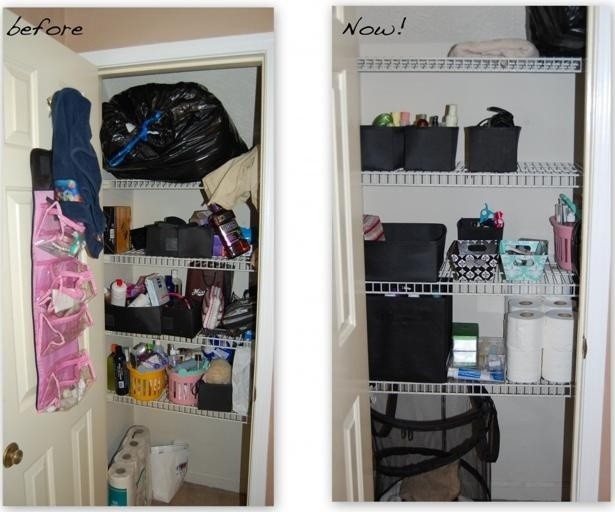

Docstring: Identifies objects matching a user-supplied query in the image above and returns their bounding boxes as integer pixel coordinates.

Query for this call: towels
[446,38,541,59]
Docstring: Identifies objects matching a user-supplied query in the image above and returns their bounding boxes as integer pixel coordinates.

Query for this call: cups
[390,110,401,125]
[401,110,410,126]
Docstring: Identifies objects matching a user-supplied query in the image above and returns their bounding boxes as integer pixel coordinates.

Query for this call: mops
[506,297,579,384]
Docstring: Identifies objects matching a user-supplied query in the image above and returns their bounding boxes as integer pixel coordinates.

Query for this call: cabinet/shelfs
[91,52,261,426]
[356,55,592,399]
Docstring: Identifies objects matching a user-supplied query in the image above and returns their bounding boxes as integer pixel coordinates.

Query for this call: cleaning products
[110,279,127,307]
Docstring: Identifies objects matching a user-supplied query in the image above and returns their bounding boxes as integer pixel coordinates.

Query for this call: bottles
[169,342,181,357]
[446,103,458,127]
[195,353,202,371]
[207,202,251,258]
[114,346,131,395]
[109,221,116,251]
[416,114,428,127]
[153,341,165,356]
[106,344,119,392]
[432,115,439,127]
[105,276,185,309]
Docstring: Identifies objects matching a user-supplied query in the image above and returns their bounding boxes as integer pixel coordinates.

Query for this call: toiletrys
[371,104,459,129]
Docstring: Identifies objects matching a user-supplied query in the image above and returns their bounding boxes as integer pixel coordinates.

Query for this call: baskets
[166,361,205,406]
[549,214,580,273]
[126,362,169,402]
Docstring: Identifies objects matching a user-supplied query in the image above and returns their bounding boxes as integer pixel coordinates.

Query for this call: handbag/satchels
[151,445,189,503]
[185,261,235,316]
